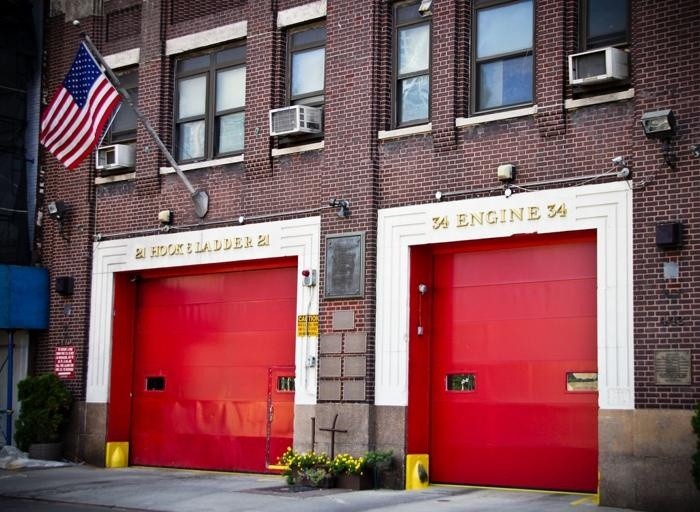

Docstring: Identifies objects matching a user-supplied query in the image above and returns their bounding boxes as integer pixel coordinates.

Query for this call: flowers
[276,446,365,481]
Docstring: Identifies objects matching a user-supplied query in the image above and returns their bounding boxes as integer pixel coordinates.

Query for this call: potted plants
[12,373,75,460]
[366,444,402,489]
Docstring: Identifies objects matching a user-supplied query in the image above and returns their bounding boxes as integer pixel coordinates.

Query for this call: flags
[39,42,124,171]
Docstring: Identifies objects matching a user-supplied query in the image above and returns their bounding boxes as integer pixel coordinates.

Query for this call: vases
[293,466,372,489]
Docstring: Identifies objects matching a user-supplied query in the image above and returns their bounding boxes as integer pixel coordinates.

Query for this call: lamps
[327,197,348,219]
[640,108,677,136]
[47,199,65,220]
